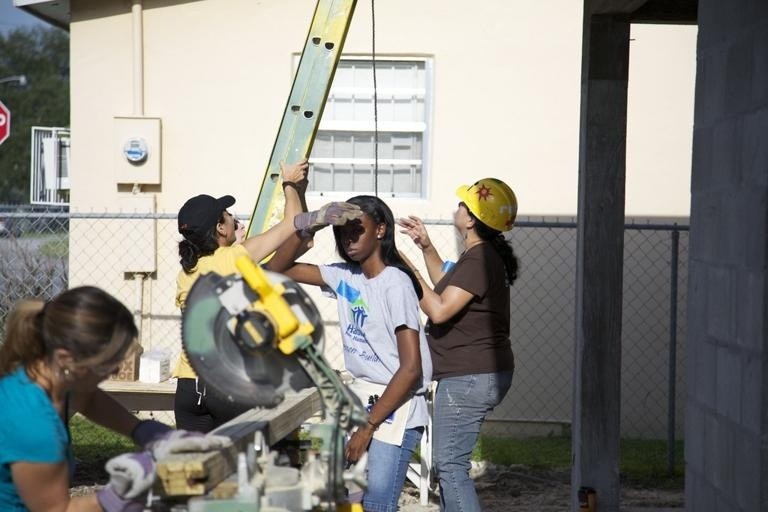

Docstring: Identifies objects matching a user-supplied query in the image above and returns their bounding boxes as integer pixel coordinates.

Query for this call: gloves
[95,451,158,512]
[129,419,235,463]
[294,202,364,241]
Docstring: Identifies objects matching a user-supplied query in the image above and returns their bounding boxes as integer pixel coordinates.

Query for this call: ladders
[245,0,358,264]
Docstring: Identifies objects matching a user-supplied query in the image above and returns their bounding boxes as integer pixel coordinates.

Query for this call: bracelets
[366,418,380,431]
[282,181,297,191]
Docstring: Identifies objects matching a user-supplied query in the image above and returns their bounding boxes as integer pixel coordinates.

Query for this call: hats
[177,194,236,238]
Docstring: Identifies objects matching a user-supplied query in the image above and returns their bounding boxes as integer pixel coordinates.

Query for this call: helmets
[454,177,518,232]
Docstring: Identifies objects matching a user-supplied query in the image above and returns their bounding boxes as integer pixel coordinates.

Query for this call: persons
[266,194,434,511]
[173,156,314,435]
[392,177,521,510]
[1,282,235,511]
